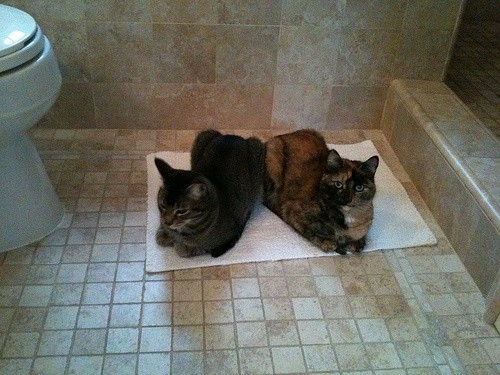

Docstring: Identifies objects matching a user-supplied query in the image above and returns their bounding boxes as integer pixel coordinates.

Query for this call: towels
[141,140,438,275]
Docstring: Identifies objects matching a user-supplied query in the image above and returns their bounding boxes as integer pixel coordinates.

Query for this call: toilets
[0,4,65,254]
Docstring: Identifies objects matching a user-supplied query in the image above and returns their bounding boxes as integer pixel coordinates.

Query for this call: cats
[154,130,266,258]
[263,128,379,255]
